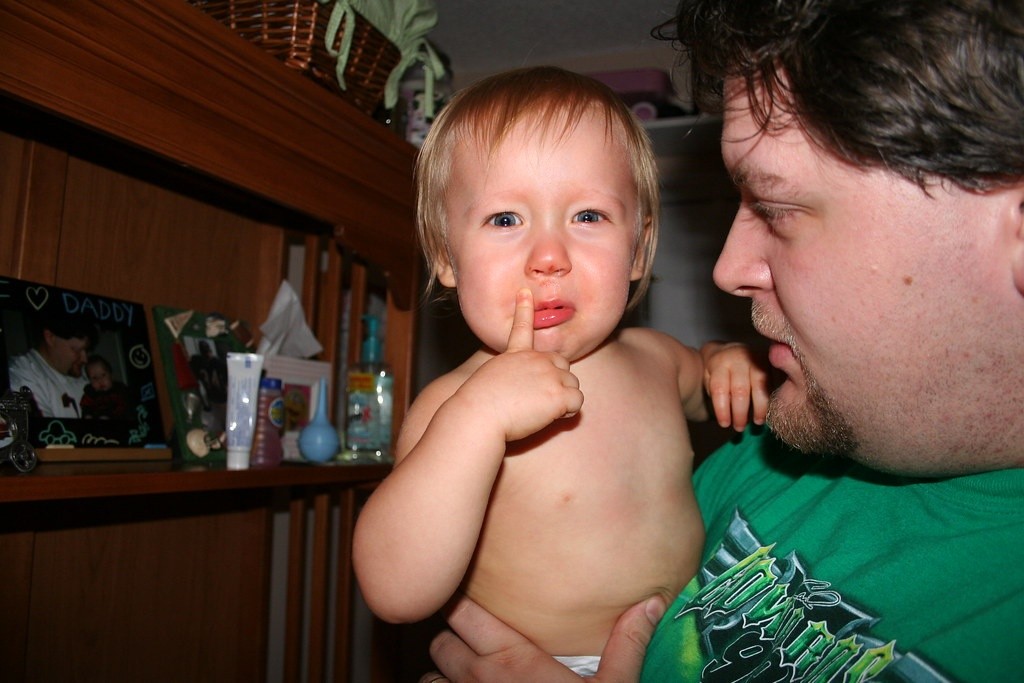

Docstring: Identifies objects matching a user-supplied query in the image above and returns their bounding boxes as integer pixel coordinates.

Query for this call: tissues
[248,278,336,462]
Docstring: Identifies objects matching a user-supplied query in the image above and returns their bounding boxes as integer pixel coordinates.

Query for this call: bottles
[249,378,283,464]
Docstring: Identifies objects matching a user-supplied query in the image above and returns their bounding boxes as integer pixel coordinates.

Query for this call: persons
[189,342,227,430]
[351,65,774,676]
[420,0,1024,683]
[7,309,137,422]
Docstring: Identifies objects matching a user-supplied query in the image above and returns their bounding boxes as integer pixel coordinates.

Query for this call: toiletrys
[225,352,266,471]
[334,312,394,464]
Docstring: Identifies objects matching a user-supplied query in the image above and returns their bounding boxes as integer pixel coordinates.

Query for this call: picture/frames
[0,273,180,461]
[266,354,332,462]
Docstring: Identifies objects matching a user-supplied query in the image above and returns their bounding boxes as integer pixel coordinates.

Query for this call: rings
[420,674,445,683]
